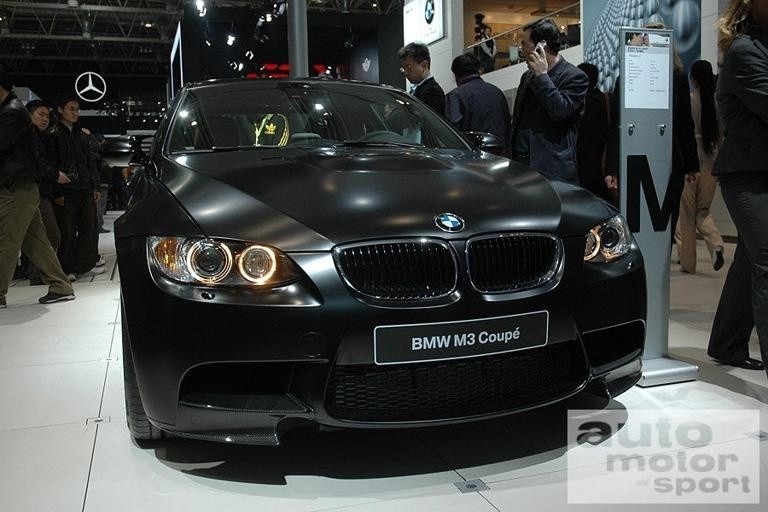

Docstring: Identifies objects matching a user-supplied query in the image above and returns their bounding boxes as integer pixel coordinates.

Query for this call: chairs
[196,115,240,150]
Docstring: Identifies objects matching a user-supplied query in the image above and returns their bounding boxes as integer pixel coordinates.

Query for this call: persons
[706,0,767,375]
[479,26,496,72]
[23,99,72,286]
[574,22,700,259]
[509,19,589,184]
[676,58,725,275]
[445,52,512,157]
[0,71,75,311]
[384,41,445,122]
[44,91,109,278]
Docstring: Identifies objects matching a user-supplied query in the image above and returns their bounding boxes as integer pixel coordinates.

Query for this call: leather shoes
[736,358,764,369]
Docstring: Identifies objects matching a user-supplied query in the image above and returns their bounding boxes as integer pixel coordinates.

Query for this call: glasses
[400,62,418,72]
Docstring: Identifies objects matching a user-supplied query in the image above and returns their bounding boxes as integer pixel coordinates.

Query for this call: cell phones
[535,42,546,55]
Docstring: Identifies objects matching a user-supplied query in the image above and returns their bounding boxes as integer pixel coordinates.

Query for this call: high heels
[679,265,688,271]
[712,246,724,270]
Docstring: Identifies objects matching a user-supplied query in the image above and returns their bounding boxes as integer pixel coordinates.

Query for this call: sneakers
[38,292,76,302]
[65,259,106,281]
[0,298,6,309]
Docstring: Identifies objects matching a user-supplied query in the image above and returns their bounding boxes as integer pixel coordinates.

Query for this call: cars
[98,135,136,168]
[102,72,658,458]
